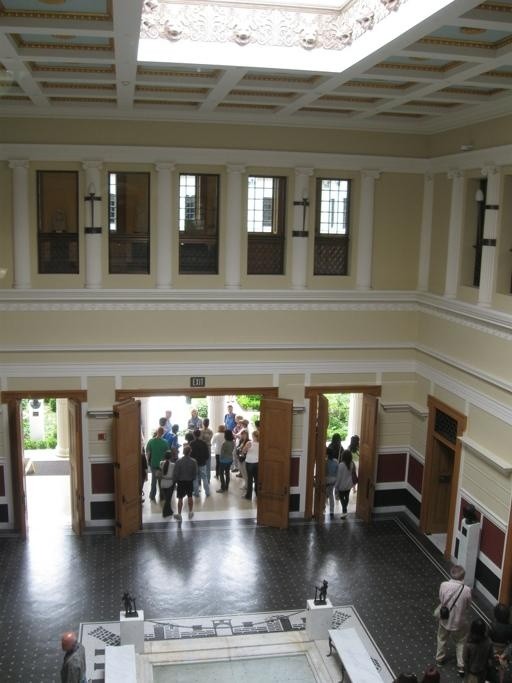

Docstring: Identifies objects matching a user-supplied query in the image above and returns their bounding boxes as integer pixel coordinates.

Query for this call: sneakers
[235,471,242,477]
[174,513,183,521]
[187,512,195,520]
[455,669,465,677]
[434,659,446,668]
[340,512,349,518]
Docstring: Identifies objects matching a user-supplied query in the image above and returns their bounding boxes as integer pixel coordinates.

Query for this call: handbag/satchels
[433,602,442,620]
[236,448,248,461]
[349,461,360,484]
[440,606,450,619]
[155,460,164,479]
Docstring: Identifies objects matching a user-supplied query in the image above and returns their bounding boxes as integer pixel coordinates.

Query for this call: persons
[122,592,134,614]
[463,504,478,524]
[392,672,418,683]
[141,405,360,520]
[435,566,472,673]
[317,581,328,602]
[486,603,512,683]
[462,619,489,683]
[422,666,440,683]
[61,632,85,683]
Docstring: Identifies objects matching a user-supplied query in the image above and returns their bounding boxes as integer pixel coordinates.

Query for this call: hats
[239,417,249,425]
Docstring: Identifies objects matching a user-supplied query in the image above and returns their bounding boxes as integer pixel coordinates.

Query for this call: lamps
[291,189,313,238]
[473,186,500,250]
[84,181,103,233]
